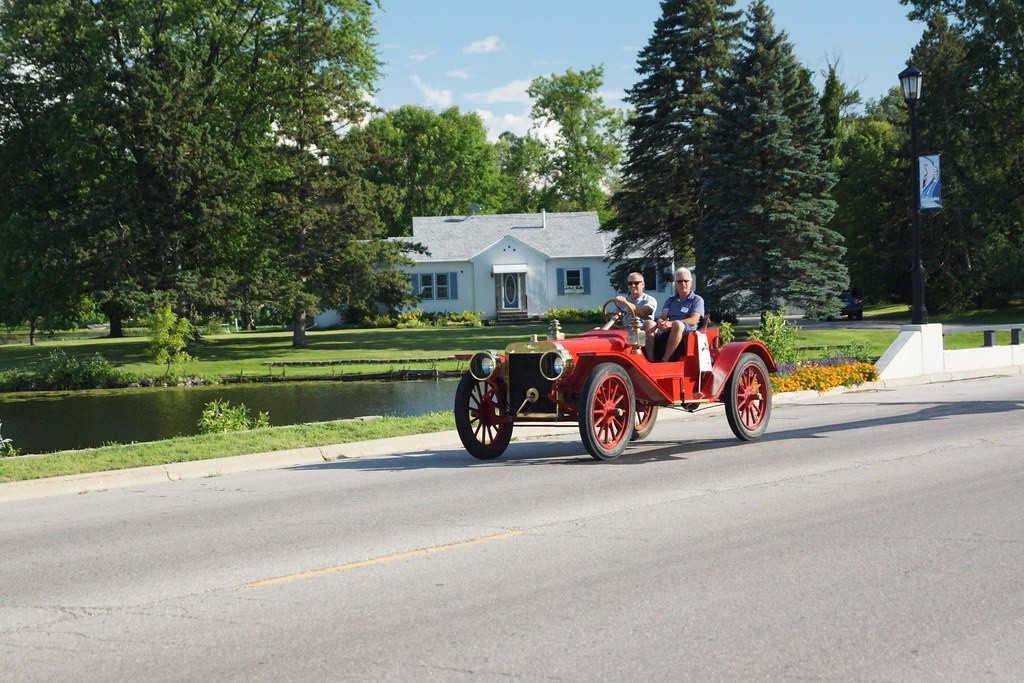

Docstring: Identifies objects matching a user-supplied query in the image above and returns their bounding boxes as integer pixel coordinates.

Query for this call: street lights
[897,58,929,326]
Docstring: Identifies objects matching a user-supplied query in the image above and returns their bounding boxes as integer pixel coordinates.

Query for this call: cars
[806,292,864,320]
[455,296,778,460]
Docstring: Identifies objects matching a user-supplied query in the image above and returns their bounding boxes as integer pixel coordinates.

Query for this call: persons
[613,272,657,330]
[644,268,705,362]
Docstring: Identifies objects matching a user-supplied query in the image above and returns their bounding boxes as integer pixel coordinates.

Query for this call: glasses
[627,281,642,285]
[676,280,690,283]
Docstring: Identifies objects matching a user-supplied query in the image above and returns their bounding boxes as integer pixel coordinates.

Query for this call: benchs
[654,313,710,363]
